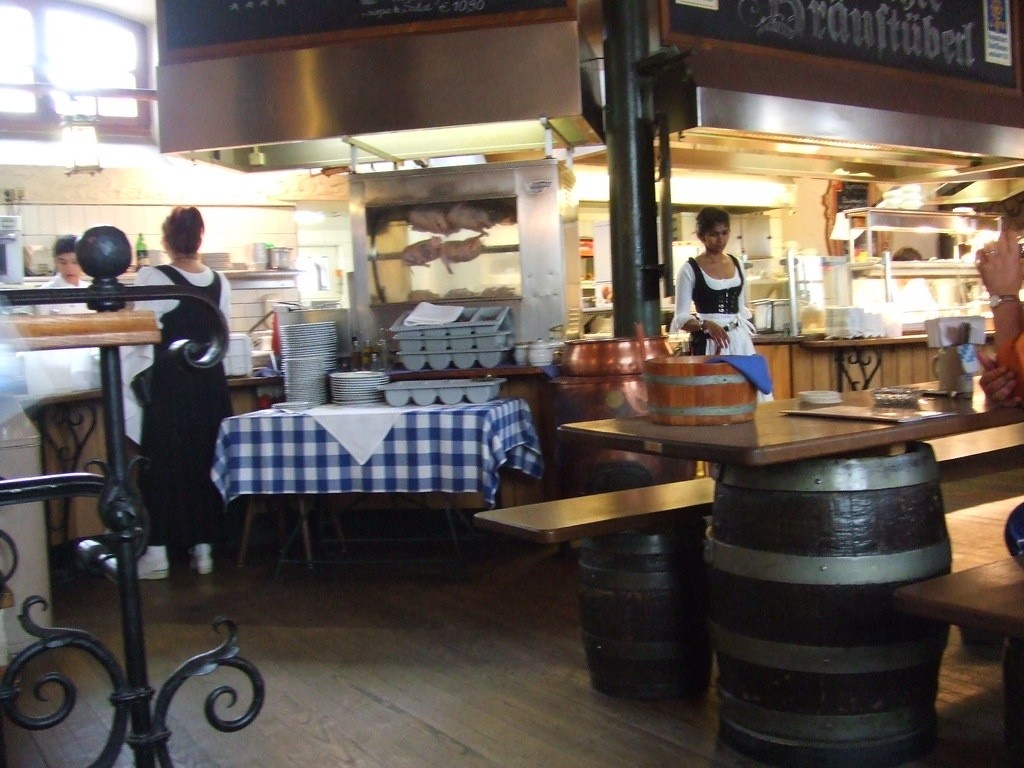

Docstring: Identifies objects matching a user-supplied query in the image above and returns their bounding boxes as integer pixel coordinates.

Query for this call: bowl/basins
[265,246,294,269]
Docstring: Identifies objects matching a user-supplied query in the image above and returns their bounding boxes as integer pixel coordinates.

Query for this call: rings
[975,256,985,270]
[985,250,997,256]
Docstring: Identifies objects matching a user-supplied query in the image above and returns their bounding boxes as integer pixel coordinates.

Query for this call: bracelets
[701,320,705,330]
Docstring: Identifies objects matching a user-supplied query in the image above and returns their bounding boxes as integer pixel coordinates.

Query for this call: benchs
[472,423,1024,551]
[885,559,1024,644]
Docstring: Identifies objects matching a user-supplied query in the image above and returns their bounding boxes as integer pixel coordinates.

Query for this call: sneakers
[190,544,213,574]
[136,546,169,579]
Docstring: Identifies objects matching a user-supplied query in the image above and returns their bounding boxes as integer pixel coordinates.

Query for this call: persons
[673,206,775,403]
[892,247,937,314]
[12,235,98,384]
[975,217,1024,412]
[132,202,231,580]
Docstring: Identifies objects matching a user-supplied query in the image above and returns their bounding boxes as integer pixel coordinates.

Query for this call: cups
[930,346,973,394]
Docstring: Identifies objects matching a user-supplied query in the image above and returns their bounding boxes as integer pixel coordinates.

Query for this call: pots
[750,298,807,333]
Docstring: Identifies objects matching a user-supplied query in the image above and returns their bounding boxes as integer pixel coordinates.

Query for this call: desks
[550,371,1024,467]
[209,396,544,573]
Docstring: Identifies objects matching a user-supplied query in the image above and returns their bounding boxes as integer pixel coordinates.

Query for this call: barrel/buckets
[704,438,952,768]
[548,335,696,500]
[642,354,757,426]
[573,515,709,699]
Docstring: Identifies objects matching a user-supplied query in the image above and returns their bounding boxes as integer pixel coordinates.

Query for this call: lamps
[58,110,104,180]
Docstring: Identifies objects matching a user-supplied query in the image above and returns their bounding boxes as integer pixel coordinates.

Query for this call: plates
[797,390,844,403]
[199,252,252,271]
[270,321,338,412]
[329,370,390,405]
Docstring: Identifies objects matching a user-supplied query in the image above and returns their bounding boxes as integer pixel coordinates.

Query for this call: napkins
[924,314,987,348]
[825,306,903,338]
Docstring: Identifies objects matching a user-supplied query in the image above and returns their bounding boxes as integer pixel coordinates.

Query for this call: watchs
[989,294,1020,309]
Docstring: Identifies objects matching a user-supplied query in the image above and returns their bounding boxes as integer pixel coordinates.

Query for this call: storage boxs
[222,331,255,380]
[753,298,808,333]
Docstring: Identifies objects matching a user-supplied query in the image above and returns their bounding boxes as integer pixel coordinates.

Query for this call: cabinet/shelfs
[846,205,1005,303]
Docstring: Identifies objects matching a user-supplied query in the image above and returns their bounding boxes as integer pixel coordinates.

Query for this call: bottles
[135,233,149,272]
[512,336,563,366]
[351,327,389,372]
